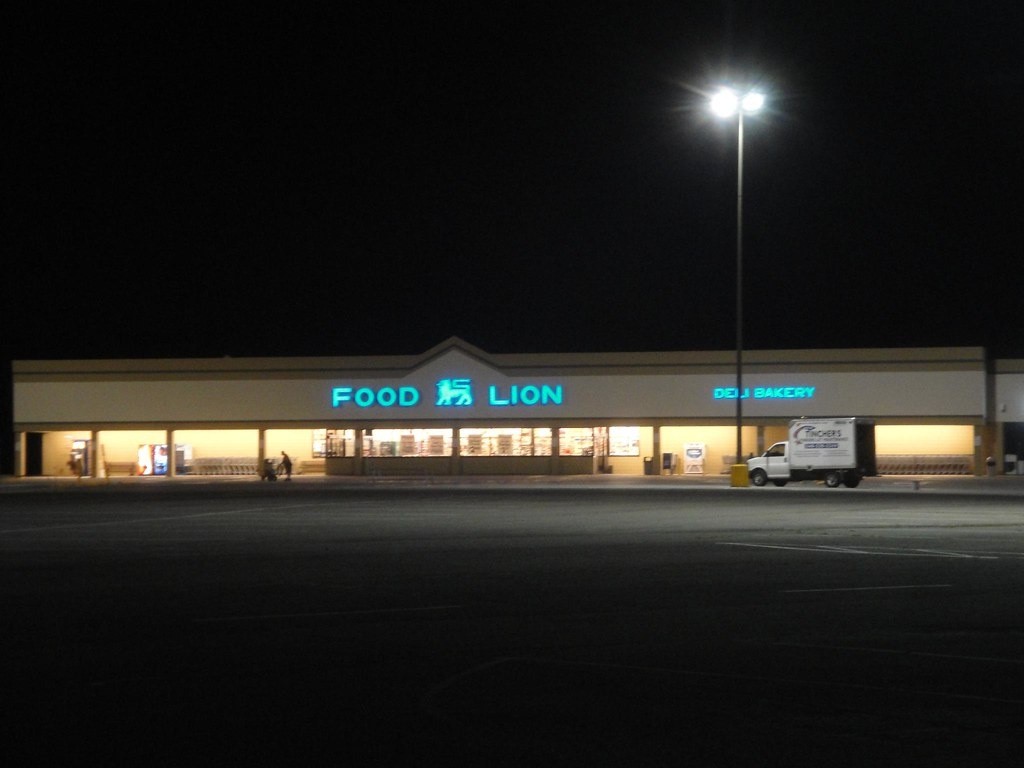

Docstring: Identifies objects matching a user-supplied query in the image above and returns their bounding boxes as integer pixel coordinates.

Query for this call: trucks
[746,417,876,488]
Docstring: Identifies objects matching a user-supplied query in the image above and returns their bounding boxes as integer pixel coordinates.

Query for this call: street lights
[708,86,768,486]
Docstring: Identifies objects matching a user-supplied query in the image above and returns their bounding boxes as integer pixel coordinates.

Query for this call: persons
[281,451,292,481]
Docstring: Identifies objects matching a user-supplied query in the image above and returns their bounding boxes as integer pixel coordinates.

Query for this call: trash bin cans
[644,457,653,475]
[263,458,275,474]
[731,464,749,487]
[985,457,996,476]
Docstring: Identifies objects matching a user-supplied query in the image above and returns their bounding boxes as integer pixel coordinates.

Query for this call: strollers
[266,463,282,482]
